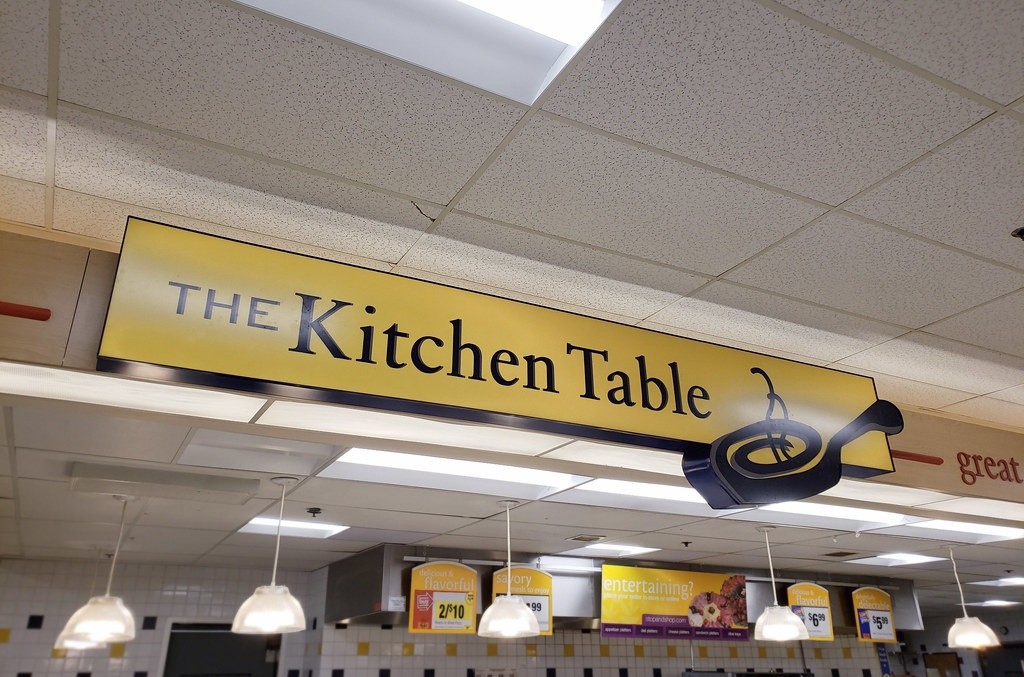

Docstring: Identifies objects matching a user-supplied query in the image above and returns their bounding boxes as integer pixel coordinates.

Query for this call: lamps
[54,494,140,651]
[939,543,1001,649]
[477,500,539,638]
[753,526,810,643]
[229,476,307,634]
